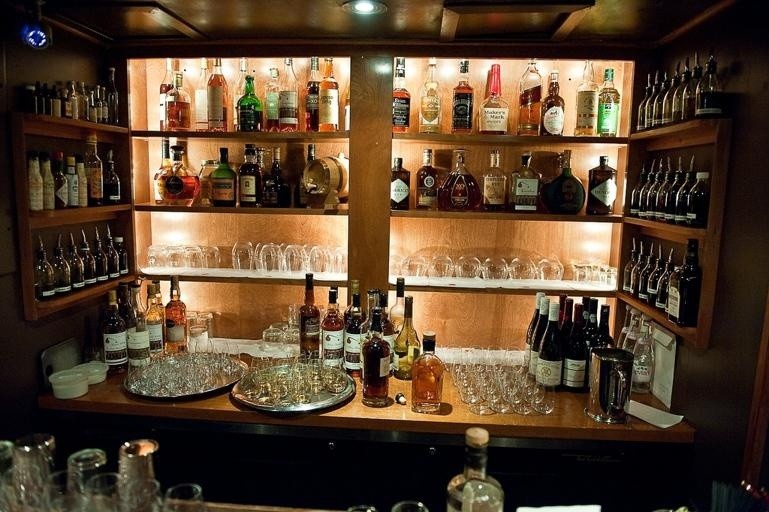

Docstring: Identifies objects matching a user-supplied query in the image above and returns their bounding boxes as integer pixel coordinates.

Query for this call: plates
[122,354,357,415]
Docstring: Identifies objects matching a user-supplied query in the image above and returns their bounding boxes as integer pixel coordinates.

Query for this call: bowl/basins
[47,363,107,401]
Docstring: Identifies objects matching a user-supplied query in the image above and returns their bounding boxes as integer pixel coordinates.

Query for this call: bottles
[391,54,622,218]
[99,274,188,375]
[152,55,350,212]
[441,426,507,512]
[299,269,443,419]
[523,285,655,395]
[25,61,128,300]
[620,47,722,328]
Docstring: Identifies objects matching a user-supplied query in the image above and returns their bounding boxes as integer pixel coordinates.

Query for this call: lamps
[372,55,394,77]
[341,0,388,19]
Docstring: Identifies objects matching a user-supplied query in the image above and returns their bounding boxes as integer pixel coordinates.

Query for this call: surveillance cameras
[19,21,53,49]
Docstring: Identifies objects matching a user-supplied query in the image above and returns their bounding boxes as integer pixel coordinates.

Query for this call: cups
[146,240,349,281]
[445,338,558,417]
[584,345,633,424]
[0,430,207,512]
[127,304,348,408]
[390,251,616,286]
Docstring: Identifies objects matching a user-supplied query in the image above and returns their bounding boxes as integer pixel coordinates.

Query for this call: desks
[59,354,697,446]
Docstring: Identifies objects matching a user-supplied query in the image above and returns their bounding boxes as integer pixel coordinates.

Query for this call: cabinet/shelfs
[617,117,731,354]
[127,44,637,348]
[10,111,135,322]
